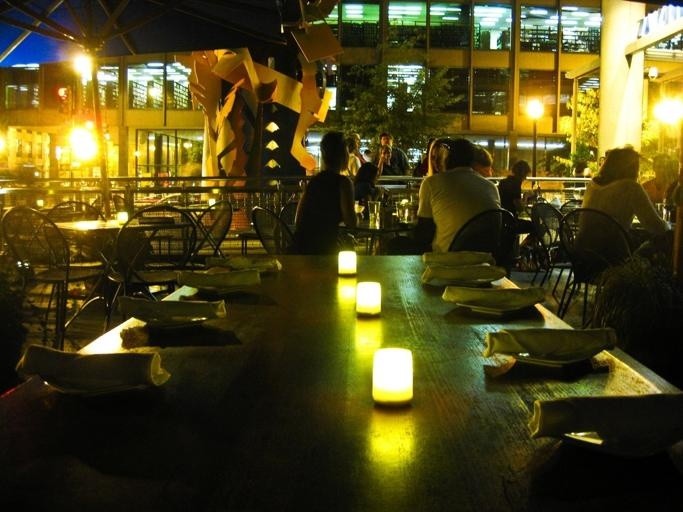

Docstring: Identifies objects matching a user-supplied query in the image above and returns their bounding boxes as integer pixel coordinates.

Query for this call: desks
[0,252,683,510]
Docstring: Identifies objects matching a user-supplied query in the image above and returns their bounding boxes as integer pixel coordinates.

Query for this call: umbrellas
[1,0,340,220]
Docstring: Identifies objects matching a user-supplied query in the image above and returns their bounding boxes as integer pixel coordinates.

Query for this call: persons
[295,131,683,262]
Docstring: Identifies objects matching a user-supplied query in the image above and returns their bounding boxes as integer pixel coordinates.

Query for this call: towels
[13,255,284,396]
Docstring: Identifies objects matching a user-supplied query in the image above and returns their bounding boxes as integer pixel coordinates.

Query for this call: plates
[42,380,149,394]
[457,302,529,316]
[512,353,594,368]
[563,429,682,446]
[466,279,496,283]
[133,314,211,326]
[189,283,248,293]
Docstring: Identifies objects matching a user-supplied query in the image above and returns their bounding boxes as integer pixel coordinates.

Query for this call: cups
[366,201,416,230]
[116,212,128,225]
[655,204,671,229]
[208,199,215,208]
